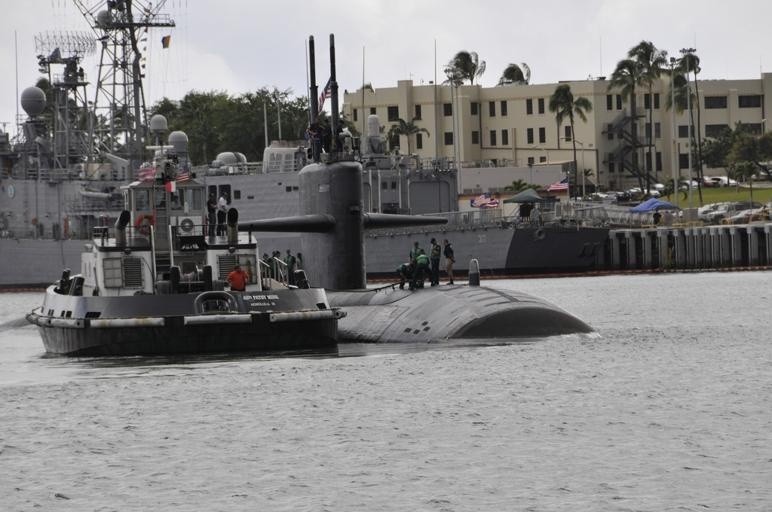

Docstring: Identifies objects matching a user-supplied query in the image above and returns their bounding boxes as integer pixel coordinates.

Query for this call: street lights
[561,137,585,197]
[530,145,549,167]
[681,48,696,209]
[669,57,682,207]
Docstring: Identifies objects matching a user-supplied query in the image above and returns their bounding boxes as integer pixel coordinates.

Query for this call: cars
[698,200,772,225]
[751,166,772,181]
[589,182,666,202]
[679,176,737,190]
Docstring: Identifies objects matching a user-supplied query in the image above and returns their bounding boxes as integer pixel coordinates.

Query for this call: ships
[27,0,347,357]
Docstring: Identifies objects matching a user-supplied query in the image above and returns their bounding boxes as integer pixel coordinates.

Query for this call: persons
[206,192,218,236]
[71,163,82,181]
[136,190,184,210]
[652,210,662,226]
[396,237,456,291]
[661,210,672,226]
[78,66,84,82]
[216,191,229,236]
[259,249,305,290]
[40,221,60,239]
[520,201,540,230]
[758,205,770,220]
[225,265,249,291]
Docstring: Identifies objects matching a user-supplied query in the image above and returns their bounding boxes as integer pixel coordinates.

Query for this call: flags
[175,169,190,183]
[137,168,156,181]
[317,77,331,114]
[470,192,499,208]
[549,175,569,190]
[165,178,177,192]
